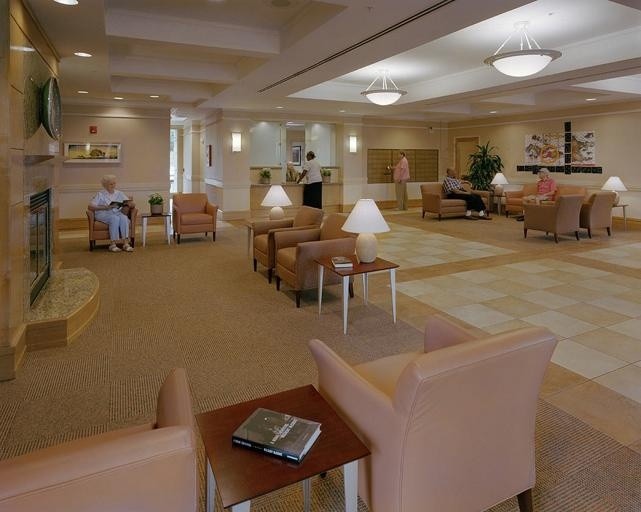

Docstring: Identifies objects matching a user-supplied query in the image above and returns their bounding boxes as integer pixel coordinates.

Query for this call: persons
[286,161,300,182]
[88,174,136,252]
[442,167,493,220]
[391,151,410,211]
[297,150,323,208]
[517,167,557,221]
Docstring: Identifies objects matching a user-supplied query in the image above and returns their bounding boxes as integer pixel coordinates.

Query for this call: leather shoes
[517,216,524,221]
[464,215,478,220]
[478,214,492,220]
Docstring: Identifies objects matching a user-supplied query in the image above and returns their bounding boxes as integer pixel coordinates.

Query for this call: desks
[613,205,629,232]
[314,255,400,335]
[195,384,371,512]
[142,211,172,247]
[491,196,506,216]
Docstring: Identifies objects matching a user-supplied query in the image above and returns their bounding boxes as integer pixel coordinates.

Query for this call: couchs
[504,183,588,218]
[420,184,490,222]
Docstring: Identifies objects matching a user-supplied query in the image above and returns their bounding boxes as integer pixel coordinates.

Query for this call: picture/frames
[64,144,121,163]
[291,146,301,166]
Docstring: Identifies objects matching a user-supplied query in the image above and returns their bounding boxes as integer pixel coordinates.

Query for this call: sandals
[123,244,134,252]
[109,245,122,252]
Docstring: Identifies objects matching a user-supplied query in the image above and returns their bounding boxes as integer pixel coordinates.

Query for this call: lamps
[482,19,562,76]
[340,199,391,263]
[600,176,628,206]
[260,184,293,220]
[491,173,509,196]
[361,68,408,105]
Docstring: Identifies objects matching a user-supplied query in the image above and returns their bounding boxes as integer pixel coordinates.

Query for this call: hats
[538,167,550,175]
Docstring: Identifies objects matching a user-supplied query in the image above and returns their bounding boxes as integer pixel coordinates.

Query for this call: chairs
[306,318,559,512]
[86,196,138,251]
[523,196,580,243]
[0,367,199,512]
[173,193,219,244]
[252,206,324,284]
[274,214,359,308]
[580,191,616,239]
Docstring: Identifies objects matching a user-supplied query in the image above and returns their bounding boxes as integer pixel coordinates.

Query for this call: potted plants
[260,168,272,184]
[322,170,331,183]
[148,192,163,213]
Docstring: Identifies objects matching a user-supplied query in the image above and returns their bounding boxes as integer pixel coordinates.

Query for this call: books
[232,407,322,464]
[109,198,131,210]
[331,256,353,268]
